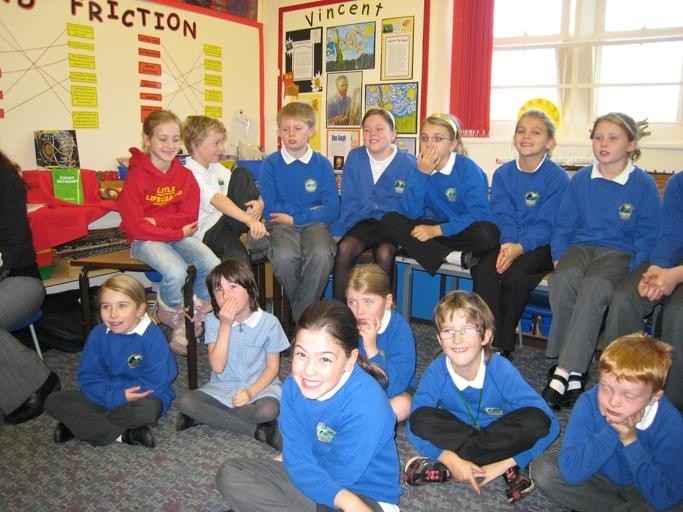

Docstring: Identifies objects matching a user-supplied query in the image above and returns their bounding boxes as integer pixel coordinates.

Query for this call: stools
[7,309,45,365]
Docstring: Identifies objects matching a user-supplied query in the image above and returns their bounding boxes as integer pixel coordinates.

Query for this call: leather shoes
[247,229,267,261]
[459,250,472,270]
[565,370,587,410]
[255,420,283,452]
[4,370,63,425]
[54,422,75,444]
[174,412,201,432]
[539,361,569,414]
[121,423,158,448]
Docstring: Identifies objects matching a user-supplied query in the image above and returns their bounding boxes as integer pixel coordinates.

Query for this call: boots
[153,286,214,355]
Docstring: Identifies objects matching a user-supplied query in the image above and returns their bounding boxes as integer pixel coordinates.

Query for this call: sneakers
[501,460,535,505]
[402,455,455,487]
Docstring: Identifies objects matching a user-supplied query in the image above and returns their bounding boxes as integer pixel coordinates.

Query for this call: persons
[332,108,417,305]
[372,113,500,277]
[174,260,291,452]
[0,151,62,424]
[402,289,561,504]
[328,75,362,126]
[116,110,224,355]
[470,111,569,363]
[182,116,270,267]
[344,262,418,423]
[257,102,340,325]
[44,274,179,448]
[541,113,662,411]
[602,170,683,417]
[530,332,682,512]
[216,299,403,512]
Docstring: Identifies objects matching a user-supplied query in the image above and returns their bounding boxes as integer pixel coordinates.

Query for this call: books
[52,168,84,204]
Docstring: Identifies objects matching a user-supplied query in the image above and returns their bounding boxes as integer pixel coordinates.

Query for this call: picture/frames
[326,71,363,129]
[326,129,360,190]
[325,21,375,71]
[365,81,418,134]
[395,137,416,156]
[380,16,415,81]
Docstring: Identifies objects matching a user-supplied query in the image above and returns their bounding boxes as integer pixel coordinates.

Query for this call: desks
[69,249,663,390]
